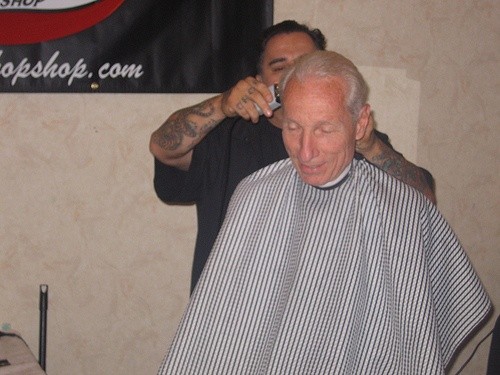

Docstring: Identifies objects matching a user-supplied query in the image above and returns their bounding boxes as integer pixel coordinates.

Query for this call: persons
[156,51,494,375]
[150,20,439,308]
